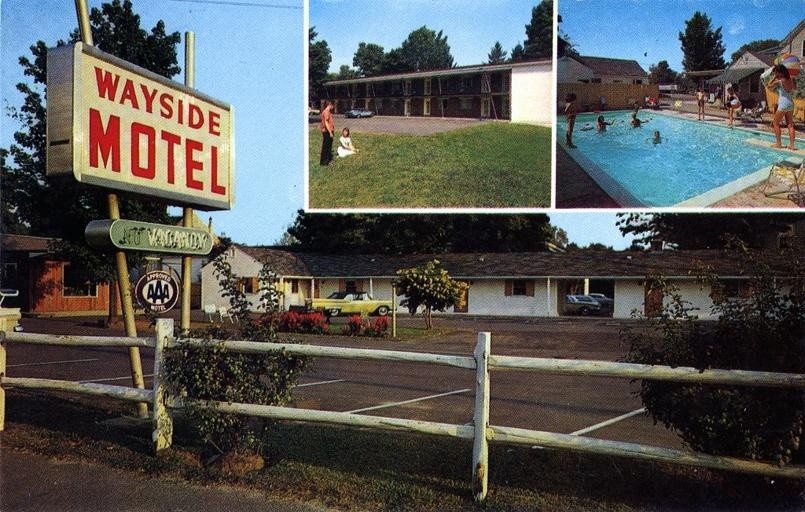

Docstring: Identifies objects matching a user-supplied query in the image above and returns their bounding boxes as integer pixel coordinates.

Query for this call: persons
[563,94,577,148]
[320,99,336,166]
[697,88,705,120]
[768,65,796,150]
[599,96,607,111]
[630,112,644,127]
[597,116,616,133]
[726,88,739,126]
[653,131,661,144]
[336,128,360,158]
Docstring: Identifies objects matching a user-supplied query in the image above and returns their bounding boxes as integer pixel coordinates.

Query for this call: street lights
[390,277,397,338]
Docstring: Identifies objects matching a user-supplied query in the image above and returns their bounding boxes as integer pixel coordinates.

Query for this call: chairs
[741,101,767,121]
[763,157,805,208]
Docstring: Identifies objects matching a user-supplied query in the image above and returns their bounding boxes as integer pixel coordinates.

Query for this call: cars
[563,293,614,316]
[309,110,322,121]
[346,109,375,119]
[304,292,397,317]
